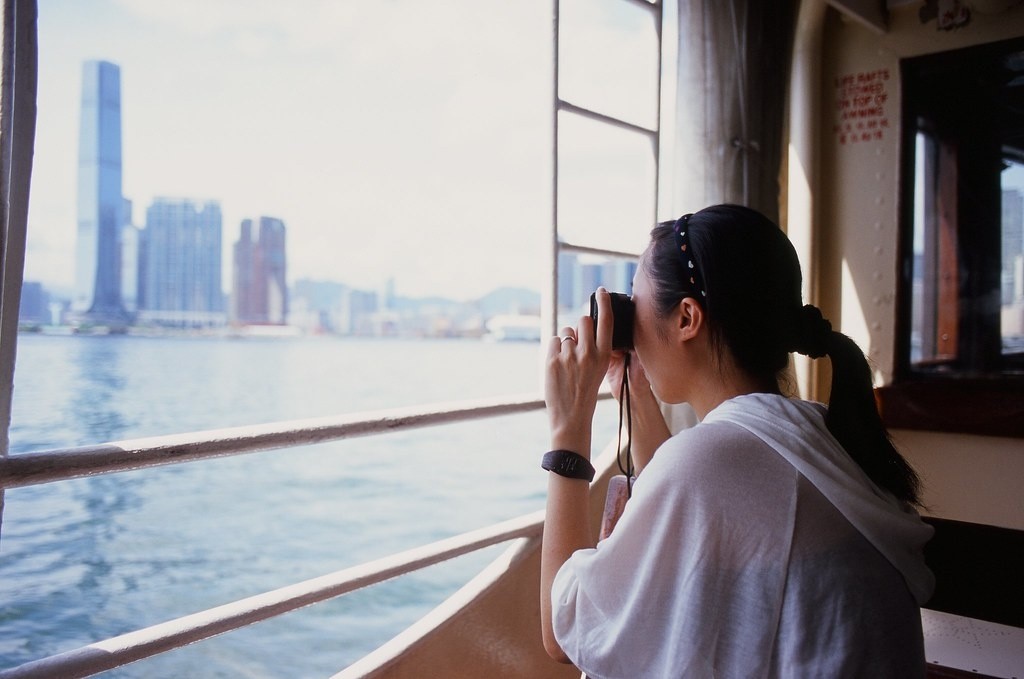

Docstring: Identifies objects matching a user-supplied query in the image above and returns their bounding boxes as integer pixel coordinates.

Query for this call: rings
[559,336,577,345]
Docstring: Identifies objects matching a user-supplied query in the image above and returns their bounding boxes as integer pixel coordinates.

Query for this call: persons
[541,202,944,676]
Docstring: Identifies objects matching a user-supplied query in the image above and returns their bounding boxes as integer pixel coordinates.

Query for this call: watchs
[542,449,597,486]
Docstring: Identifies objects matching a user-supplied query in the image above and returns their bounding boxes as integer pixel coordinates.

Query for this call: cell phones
[590,292,634,349]
